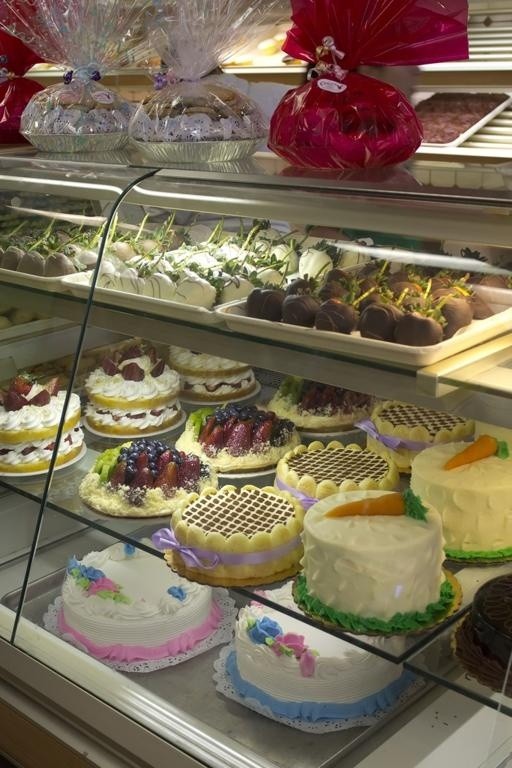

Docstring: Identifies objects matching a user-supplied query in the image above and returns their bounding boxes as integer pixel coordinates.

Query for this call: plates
[81,409,190,440]
[141,156,277,176]
[130,137,270,162]
[178,381,262,407]
[0,441,89,477]
[24,129,130,155]
[214,465,277,479]
[31,150,133,166]
[299,426,362,438]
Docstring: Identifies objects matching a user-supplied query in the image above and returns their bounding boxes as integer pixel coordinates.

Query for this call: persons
[279,62,438,274]
[143,43,295,239]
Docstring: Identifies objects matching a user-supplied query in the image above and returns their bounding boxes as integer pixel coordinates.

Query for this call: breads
[0,22,510,167]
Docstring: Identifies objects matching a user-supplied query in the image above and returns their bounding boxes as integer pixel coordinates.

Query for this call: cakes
[0,342,512,736]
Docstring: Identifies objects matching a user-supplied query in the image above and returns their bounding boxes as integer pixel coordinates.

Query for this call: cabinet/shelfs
[1,146,511,768]
[25,0,512,163]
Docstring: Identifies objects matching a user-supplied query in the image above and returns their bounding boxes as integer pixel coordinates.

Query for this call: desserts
[0,225,492,347]
